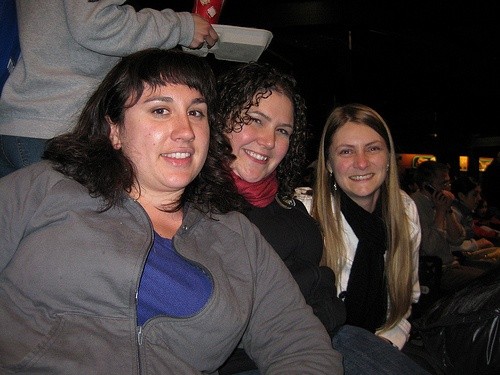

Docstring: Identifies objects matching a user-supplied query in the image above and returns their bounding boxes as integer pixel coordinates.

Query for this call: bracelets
[387,340,398,350]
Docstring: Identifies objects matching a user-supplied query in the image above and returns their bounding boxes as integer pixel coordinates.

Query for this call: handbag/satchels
[416,307,500,375]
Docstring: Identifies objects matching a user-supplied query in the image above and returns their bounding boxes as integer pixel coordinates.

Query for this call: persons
[0,0,219,174]
[0,49,344,374]
[395,145,500,313]
[204,63,428,375]
[293,103,423,351]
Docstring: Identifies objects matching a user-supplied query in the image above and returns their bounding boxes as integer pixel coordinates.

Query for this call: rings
[203,38,206,43]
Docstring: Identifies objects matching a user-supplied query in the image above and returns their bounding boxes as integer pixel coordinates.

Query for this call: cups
[191,0,224,23]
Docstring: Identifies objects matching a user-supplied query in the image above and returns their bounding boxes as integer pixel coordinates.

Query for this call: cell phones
[423,183,446,201]
